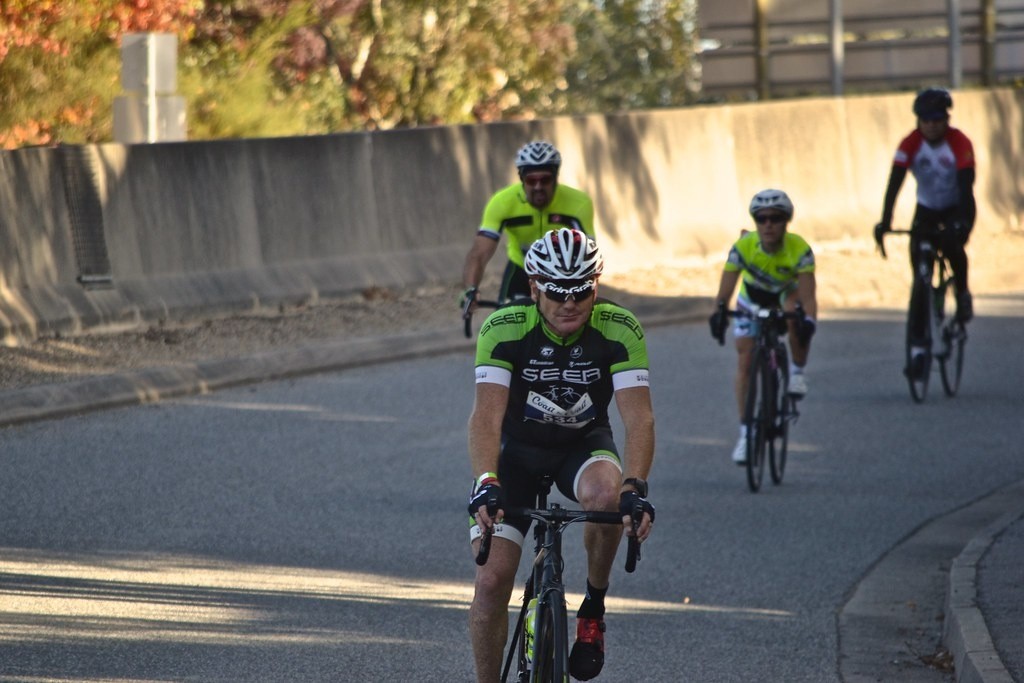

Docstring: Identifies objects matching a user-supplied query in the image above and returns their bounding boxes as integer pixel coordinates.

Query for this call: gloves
[953,224,968,242]
[875,222,888,243]
[708,313,728,338]
[798,319,815,348]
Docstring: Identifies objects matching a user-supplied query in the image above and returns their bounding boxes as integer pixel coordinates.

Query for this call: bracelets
[477,472,498,485]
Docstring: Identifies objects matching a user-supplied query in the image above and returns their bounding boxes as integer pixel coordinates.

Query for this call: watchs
[623,478,648,498]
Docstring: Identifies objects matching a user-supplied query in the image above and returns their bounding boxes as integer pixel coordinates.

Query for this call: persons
[458,142,596,314]
[881,87,977,376]
[709,189,817,461]
[466,228,655,683]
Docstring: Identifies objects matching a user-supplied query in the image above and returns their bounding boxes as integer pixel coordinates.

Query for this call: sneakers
[570,600,606,680]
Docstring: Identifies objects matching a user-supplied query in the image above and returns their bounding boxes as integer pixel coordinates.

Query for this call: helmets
[524,228,604,282]
[516,142,561,167]
[913,90,952,112]
[749,189,794,217]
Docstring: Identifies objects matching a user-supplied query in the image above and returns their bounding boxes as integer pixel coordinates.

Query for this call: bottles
[526,595,538,661]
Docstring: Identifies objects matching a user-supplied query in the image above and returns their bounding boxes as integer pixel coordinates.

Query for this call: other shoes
[903,353,924,377]
[733,439,749,461]
[955,300,972,322]
[787,374,807,394]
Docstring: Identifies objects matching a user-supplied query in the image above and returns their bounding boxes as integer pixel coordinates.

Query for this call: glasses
[522,174,554,186]
[535,278,596,302]
[753,213,784,223]
[919,112,943,121]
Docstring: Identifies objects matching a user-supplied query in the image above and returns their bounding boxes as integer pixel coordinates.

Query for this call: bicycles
[880,229,969,404]
[475,474,642,683]
[462,287,534,338]
[716,300,806,493]
